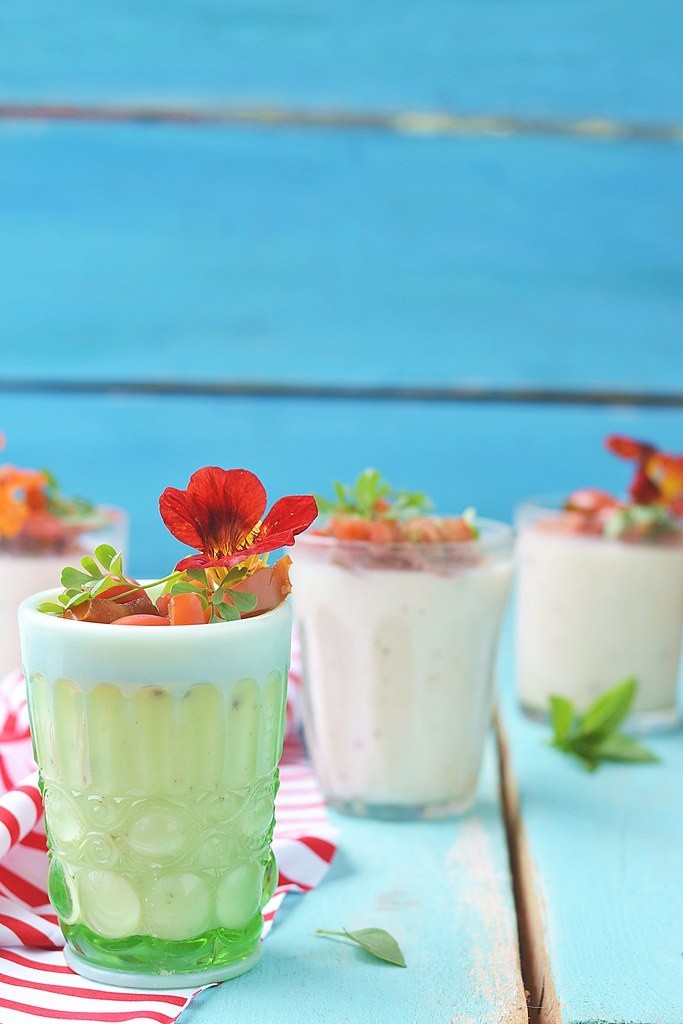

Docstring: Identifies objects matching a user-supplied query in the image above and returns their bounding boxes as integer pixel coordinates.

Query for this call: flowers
[159,464,319,573]
[603,433,683,520]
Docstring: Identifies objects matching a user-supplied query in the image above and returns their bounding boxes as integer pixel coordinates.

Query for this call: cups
[510,508,683,730]
[0,505,141,723]
[278,513,510,824]
[18,581,294,988]
